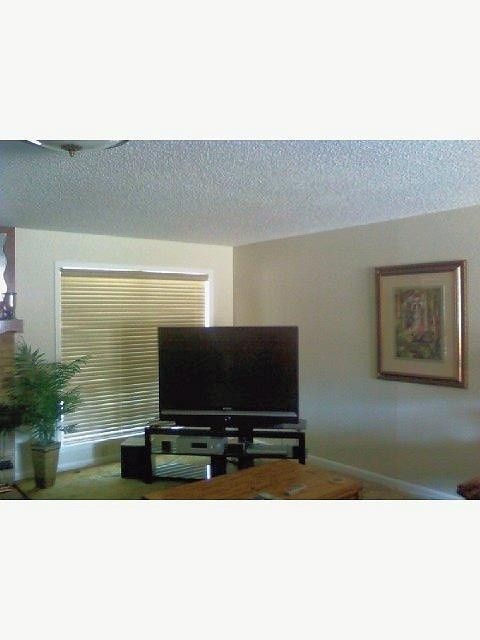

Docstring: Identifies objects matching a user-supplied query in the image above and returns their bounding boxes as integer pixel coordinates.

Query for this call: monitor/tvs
[157,325,300,430]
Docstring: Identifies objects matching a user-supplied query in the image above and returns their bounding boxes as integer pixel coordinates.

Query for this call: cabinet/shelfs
[143,426,306,484]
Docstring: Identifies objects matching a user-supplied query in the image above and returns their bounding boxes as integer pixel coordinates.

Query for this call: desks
[143,458,363,500]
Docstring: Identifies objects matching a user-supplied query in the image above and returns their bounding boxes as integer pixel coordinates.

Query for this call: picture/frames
[375,259,469,389]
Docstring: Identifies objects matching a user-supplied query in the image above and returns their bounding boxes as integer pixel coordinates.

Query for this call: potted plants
[0,338,92,490]
